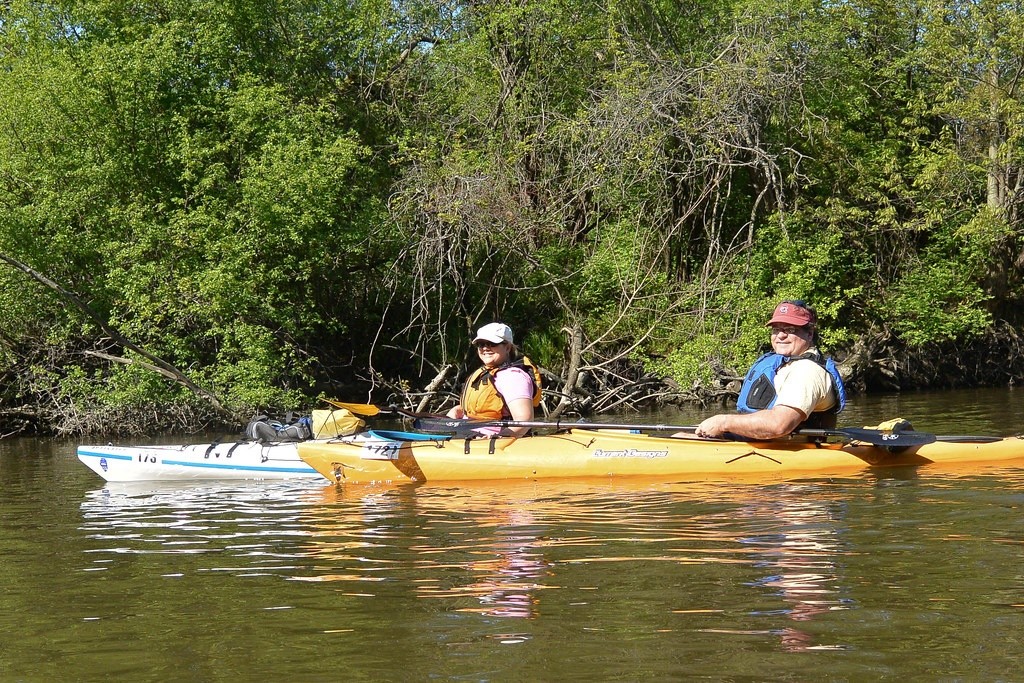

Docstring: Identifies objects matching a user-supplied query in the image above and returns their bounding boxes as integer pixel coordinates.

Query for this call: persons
[671,300,845,442]
[444,322,542,438]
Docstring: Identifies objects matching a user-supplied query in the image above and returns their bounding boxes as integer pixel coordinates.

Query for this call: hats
[765,300,818,326]
[472,322,513,344]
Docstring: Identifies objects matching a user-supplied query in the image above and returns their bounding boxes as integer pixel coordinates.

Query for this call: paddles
[321,395,446,425]
[414,419,940,447]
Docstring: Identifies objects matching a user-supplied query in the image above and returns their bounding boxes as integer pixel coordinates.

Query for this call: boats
[76,429,643,489]
[297,427,1022,491]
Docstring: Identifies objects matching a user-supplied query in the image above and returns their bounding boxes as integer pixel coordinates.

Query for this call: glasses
[474,340,506,348]
[768,325,804,334]
